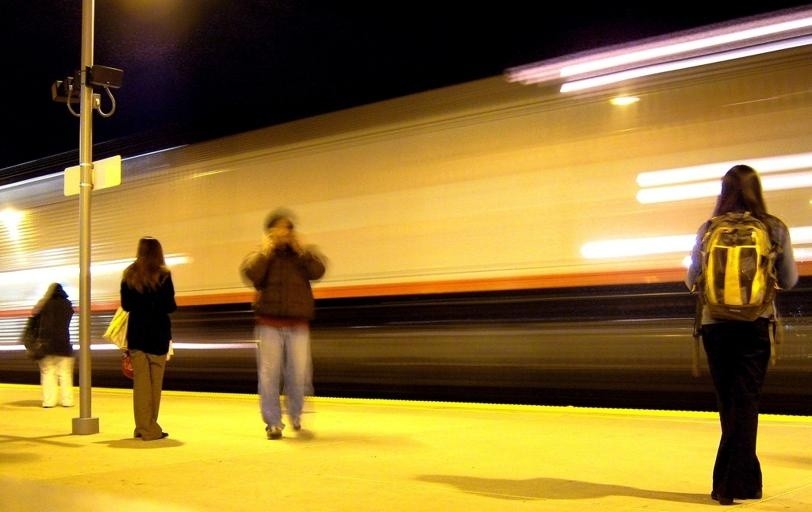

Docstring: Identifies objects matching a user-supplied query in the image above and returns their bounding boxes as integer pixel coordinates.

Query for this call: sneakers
[711,489,762,504]
[265,423,285,439]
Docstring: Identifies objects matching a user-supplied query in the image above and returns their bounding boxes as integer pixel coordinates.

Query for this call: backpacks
[690,211,783,322]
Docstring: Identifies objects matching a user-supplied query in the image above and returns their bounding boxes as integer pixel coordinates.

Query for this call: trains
[0,4,812,415]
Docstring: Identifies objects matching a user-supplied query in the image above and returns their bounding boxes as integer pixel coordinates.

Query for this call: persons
[32,282,76,408]
[683,164,797,501]
[238,204,328,438]
[120,235,176,441]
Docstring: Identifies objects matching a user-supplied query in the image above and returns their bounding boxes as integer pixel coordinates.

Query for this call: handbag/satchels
[102,306,130,352]
[120,352,134,380]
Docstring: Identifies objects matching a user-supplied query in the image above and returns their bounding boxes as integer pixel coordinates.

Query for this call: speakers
[51,80,81,102]
[87,64,125,90]
